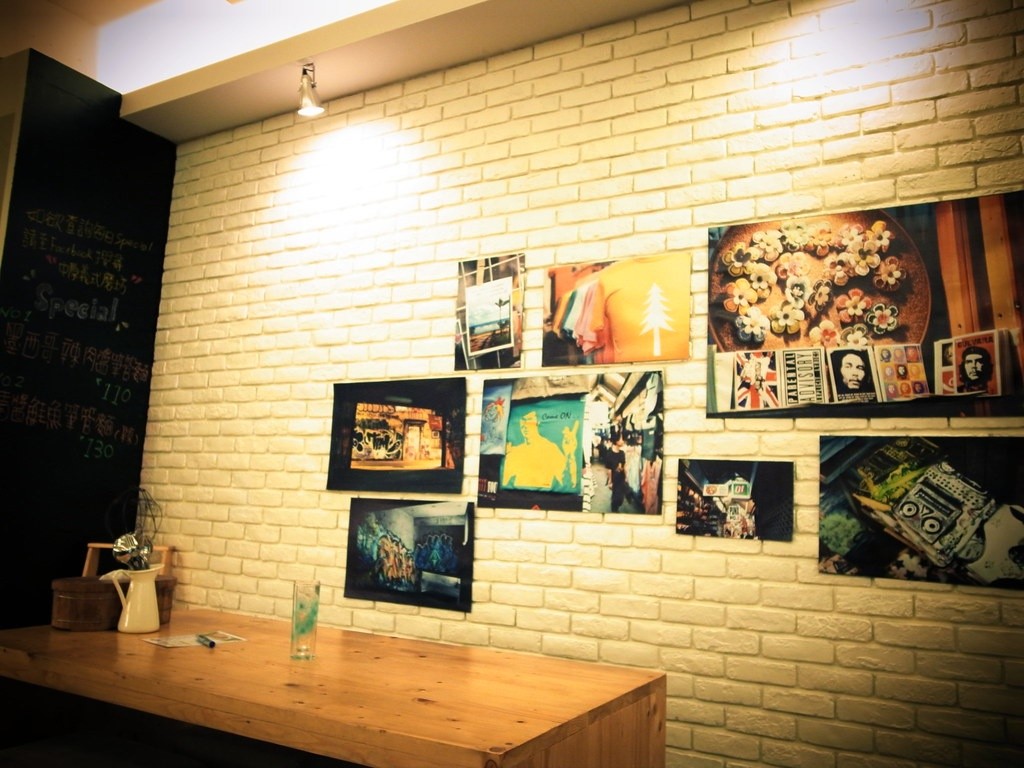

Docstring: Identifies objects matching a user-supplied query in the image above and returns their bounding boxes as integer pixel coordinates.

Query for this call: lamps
[297,62,326,119]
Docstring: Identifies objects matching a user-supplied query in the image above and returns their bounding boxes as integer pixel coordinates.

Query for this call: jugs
[112,562,164,634]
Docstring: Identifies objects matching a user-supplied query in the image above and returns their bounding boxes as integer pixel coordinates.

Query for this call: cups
[291,579,321,659]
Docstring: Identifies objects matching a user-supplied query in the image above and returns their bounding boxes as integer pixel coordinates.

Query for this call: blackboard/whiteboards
[0,76,181,628]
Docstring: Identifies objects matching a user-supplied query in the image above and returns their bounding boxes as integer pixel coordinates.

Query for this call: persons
[502,408,580,492]
[834,351,871,399]
[604,432,626,513]
[957,346,994,396]
[881,345,927,398]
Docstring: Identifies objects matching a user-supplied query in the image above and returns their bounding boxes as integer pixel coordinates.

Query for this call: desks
[0,608,668,768]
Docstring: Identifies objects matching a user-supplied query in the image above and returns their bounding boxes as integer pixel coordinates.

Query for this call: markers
[195,631,215,648]
